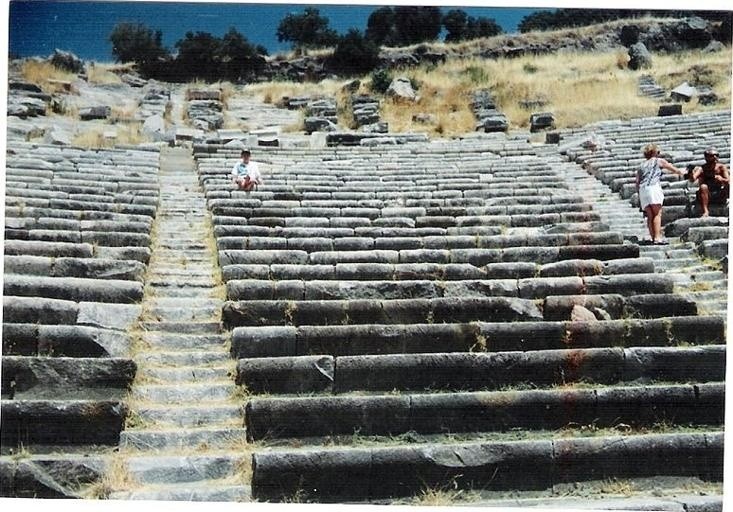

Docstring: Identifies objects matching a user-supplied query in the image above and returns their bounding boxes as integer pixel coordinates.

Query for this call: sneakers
[651,240,669,244]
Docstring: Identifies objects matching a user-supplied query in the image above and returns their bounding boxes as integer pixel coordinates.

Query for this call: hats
[242,148,250,153]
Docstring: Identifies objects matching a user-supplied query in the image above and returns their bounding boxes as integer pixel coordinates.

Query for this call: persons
[633,141,689,246]
[684,146,729,218]
[227,147,264,193]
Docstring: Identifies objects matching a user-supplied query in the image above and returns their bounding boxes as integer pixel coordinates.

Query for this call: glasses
[657,151,660,155]
[710,154,719,157]
